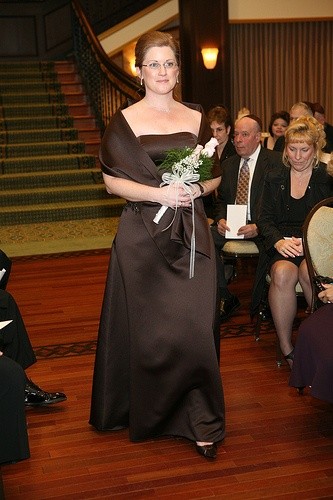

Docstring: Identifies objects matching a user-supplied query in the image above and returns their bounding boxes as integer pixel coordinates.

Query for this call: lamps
[200,47,219,69]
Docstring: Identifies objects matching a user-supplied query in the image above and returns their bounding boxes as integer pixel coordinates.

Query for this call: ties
[236,157,250,223]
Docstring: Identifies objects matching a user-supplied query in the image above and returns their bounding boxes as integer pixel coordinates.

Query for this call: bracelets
[197,182,204,196]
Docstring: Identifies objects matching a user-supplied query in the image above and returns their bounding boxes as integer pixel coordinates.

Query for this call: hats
[243,114,263,131]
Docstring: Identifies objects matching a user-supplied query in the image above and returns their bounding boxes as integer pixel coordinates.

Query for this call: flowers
[153,137,219,224]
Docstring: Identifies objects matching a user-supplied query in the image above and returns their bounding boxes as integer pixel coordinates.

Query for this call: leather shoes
[23,379,67,408]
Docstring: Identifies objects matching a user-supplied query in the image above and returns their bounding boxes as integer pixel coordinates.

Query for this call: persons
[313,103,333,153]
[89,31,228,459]
[262,112,290,152]
[210,115,282,320]
[204,104,236,225]
[290,280,333,402]
[0,250,67,466]
[272,101,313,153]
[257,115,333,370]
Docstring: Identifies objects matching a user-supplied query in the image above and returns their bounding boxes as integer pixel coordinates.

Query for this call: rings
[282,248,285,252]
[328,301,331,303]
[181,201,183,207]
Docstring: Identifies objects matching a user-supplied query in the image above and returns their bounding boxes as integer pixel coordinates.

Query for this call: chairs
[303,198,333,317]
[224,241,268,341]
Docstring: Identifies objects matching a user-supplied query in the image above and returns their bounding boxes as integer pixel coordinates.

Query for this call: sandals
[279,340,296,369]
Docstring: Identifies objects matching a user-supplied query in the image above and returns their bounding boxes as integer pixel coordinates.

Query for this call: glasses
[141,61,179,70]
[212,126,226,134]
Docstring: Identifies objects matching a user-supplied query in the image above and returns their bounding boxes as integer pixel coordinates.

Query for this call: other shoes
[218,294,241,321]
[194,439,218,459]
[258,304,272,320]
